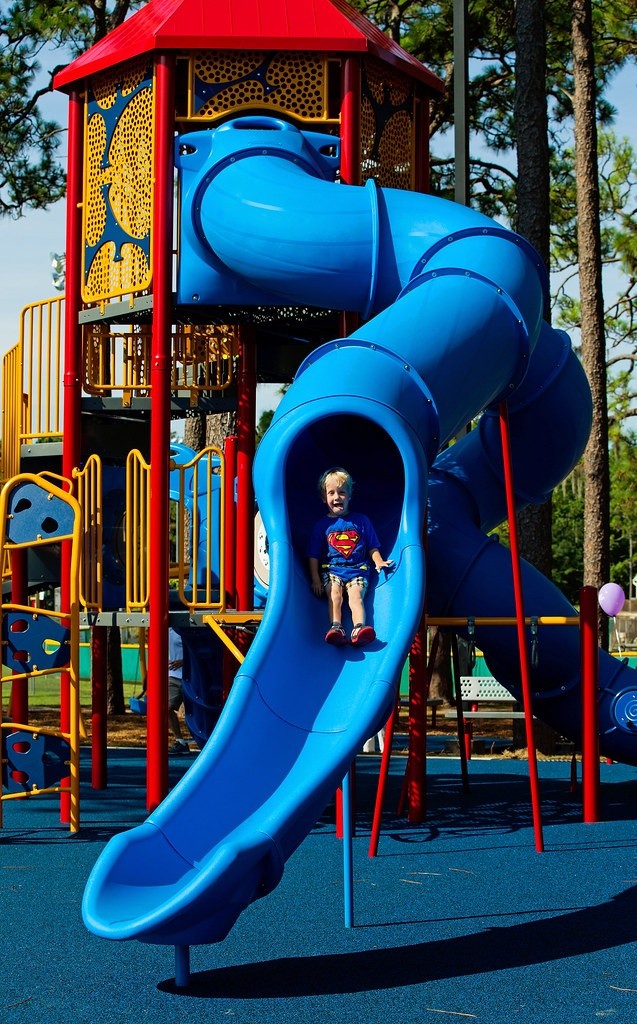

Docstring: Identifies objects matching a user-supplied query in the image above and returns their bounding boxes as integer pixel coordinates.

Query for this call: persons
[307,466,394,647]
[168,627,190,754]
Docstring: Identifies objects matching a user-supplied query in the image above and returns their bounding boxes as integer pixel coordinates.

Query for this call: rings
[174,666,175,667]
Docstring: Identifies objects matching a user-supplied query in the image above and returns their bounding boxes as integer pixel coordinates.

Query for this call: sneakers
[167,741,190,755]
[324,627,347,648]
[349,624,376,647]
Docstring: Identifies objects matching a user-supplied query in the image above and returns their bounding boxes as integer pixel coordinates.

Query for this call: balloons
[598,583,625,617]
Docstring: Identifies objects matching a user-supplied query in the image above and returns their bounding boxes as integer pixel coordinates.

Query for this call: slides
[174,625,224,751]
[80,122,544,938]
[420,323,636,768]
